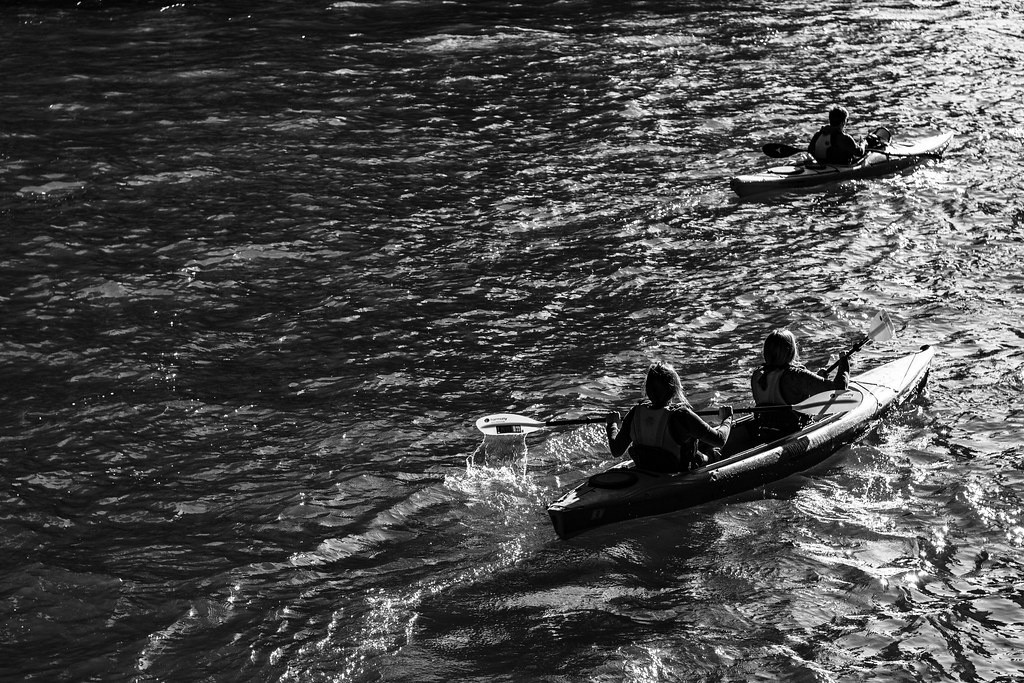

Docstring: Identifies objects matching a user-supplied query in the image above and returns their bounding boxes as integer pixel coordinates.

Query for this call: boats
[545,346,939,549]
[729,130,966,202]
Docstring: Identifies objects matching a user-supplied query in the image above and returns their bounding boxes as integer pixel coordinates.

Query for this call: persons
[606,362,733,475]
[808,105,869,168]
[751,328,851,442]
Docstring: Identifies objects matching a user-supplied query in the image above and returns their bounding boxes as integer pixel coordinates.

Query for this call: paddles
[474,389,864,437]
[761,142,944,161]
[826,309,896,375]
